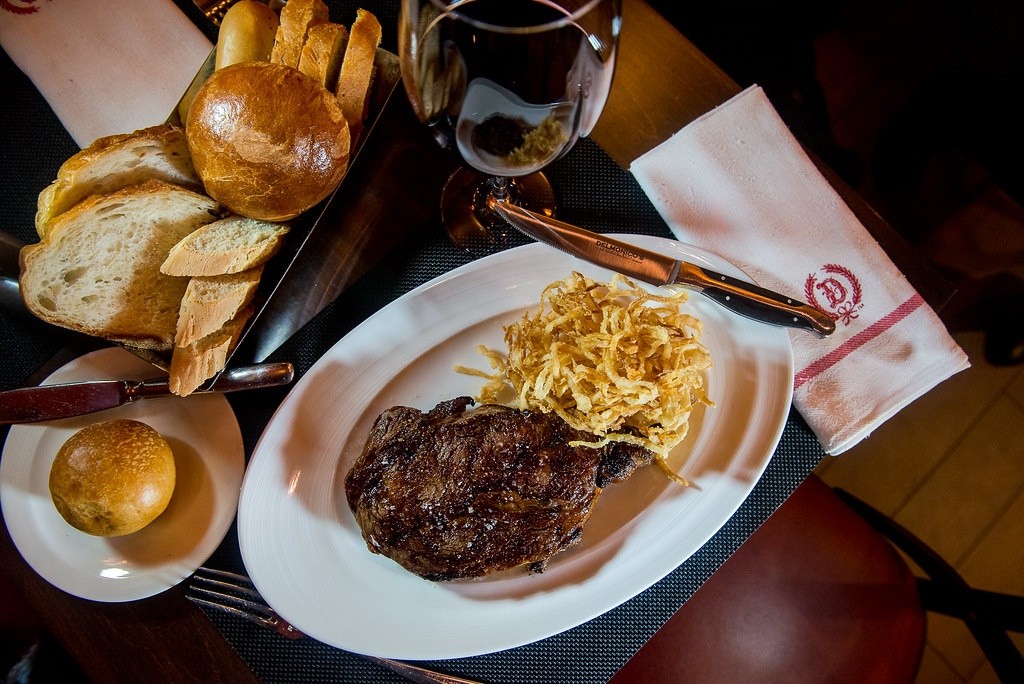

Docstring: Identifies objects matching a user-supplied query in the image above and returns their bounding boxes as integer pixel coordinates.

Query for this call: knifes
[492,197,839,340]
[0,364,297,423]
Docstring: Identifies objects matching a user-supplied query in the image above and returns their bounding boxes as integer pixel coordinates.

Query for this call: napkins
[629,80,975,460]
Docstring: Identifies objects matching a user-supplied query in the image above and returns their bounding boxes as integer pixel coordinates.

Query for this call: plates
[236,228,797,659]
[0,342,244,604]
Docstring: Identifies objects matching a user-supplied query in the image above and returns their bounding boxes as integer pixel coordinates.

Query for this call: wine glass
[395,0,622,256]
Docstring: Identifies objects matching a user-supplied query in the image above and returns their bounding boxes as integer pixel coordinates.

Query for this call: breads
[18,0,381,398]
[47,419,177,538]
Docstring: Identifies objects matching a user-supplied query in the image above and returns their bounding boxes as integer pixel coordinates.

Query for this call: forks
[181,566,485,684]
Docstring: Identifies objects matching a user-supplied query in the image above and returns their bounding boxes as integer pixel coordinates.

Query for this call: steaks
[341,395,658,585]
